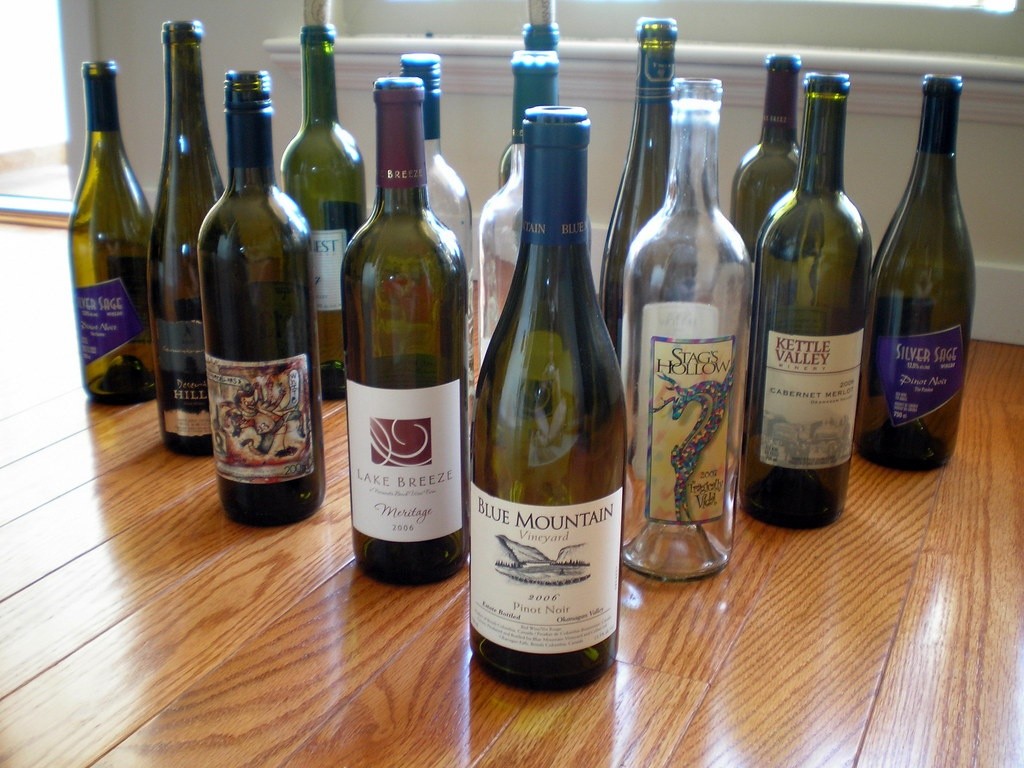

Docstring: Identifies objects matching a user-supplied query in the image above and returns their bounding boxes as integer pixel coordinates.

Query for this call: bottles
[621,76,754,581]
[67,61,157,407]
[600,18,679,358]
[498,0,560,187]
[147,20,225,458]
[738,72,872,530]
[195,68,327,528]
[400,53,473,444]
[729,54,801,455]
[479,50,560,365]
[282,0,366,401]
[468,105,627,695]
[341,76,469,586]
[852,73,977,472]
